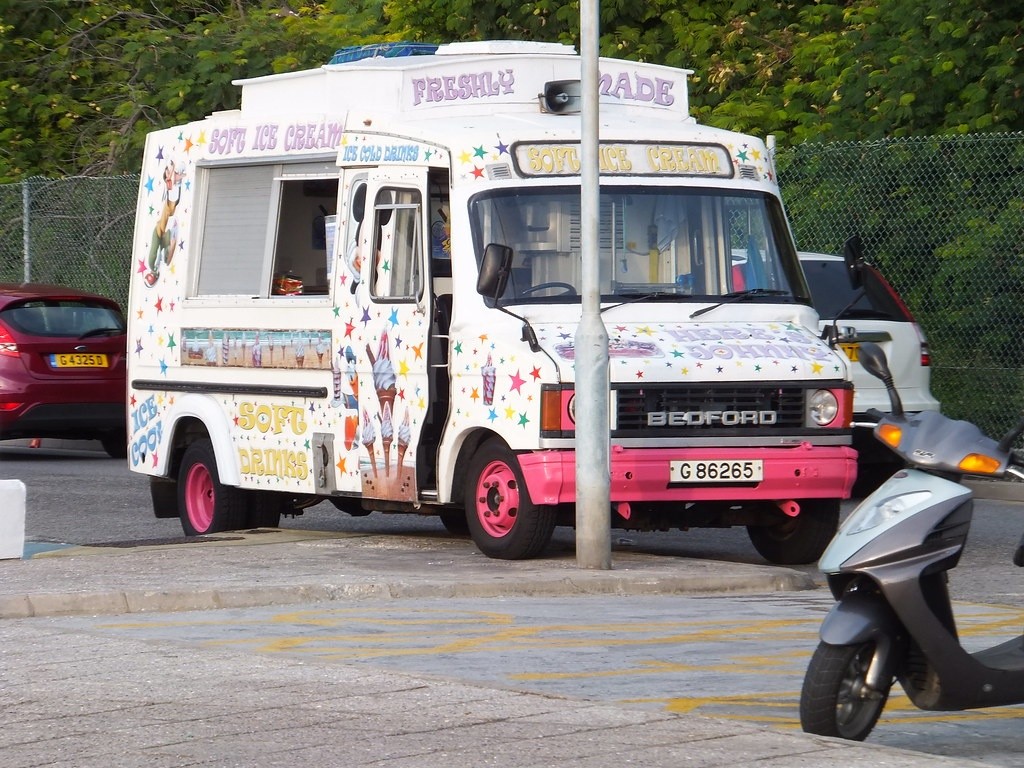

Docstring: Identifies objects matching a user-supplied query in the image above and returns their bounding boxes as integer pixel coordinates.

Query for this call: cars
[0,286,126,463]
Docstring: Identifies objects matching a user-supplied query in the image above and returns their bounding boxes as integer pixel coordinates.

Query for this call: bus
[125,40,860,567]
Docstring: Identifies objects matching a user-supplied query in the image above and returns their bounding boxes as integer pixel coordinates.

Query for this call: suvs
[710,251,942,494]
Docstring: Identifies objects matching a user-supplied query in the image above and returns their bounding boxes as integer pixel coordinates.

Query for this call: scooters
[798,344,1024,742]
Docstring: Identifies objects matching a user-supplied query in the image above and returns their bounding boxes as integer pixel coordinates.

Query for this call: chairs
[15,310,41,333]
